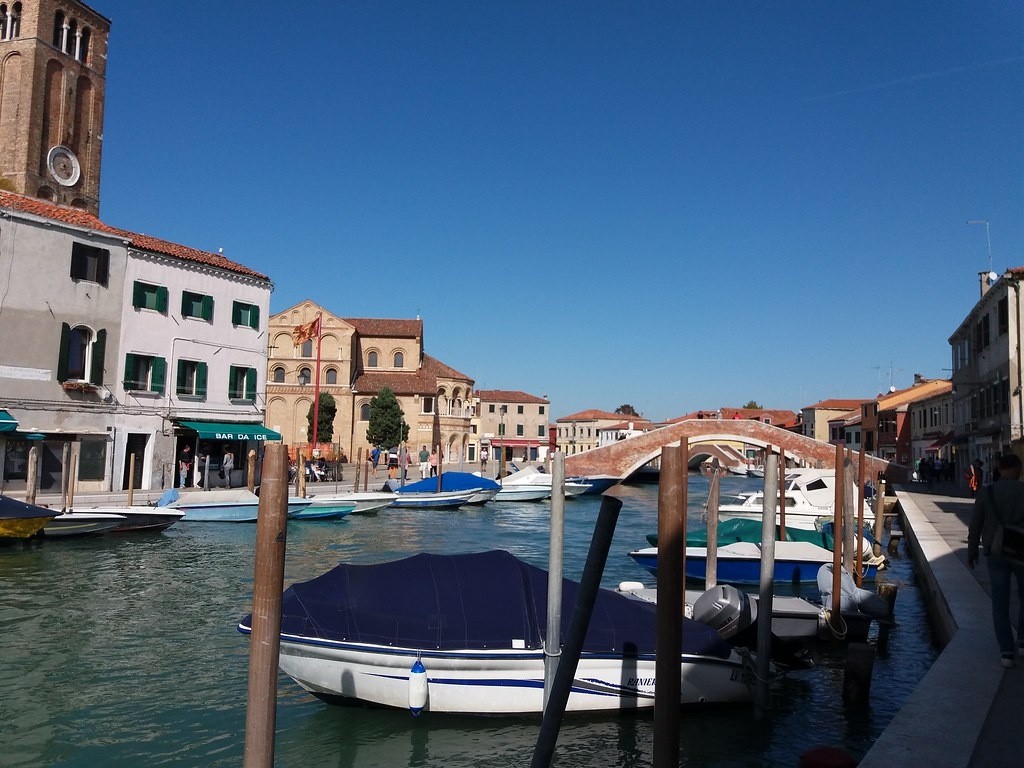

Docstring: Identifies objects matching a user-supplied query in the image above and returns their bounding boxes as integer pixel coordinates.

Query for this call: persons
[418,445,437,479]
[733,412,741,419]
[481,447,487,471]
[288,456,329,482]
[223,445,234,489]
[179,445,205,489]
[388,446,400,478]
[370,446,381,478]
[883,456,896,462]
[697,412,703,419]
[968,459,984,498]
[523,450,528,462]
[915,456,955,483]
[968,454,1024,667]
[618,433,626,440]
[399,449,412,481]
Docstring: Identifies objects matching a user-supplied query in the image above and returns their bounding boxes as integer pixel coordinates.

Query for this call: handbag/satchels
[368,455,375,461]
[990,524,1024,566]
[218,467,225,479]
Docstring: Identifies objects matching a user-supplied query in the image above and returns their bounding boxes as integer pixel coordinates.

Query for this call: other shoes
[1016,648,1024,656]
[1001,654,1014,668]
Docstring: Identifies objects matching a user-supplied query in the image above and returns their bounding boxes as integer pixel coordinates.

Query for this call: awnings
[0,410,19,431]
[176,421,281,440]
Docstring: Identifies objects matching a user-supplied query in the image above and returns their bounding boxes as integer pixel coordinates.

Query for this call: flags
[293,318,319,347]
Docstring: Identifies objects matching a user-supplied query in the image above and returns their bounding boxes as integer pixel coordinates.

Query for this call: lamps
[879,421,896,426]
[952,382,989,393]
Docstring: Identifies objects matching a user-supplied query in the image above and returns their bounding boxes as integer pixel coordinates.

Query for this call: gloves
[967,551,978,568]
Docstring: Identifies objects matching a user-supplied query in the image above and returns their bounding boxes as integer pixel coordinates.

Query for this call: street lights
[498,407,506,478]
[572,419,576,454]
[399,416,405,456]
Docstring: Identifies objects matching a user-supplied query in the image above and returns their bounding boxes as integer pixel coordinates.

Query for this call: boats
[645,518,886,570]
[147,490,313,523]
[626,541,888,584]
[491,485,552,502]
[613,581,873,655]
[294,500,357,520]
[42,513,127,539]
[237,549,787,716]
[306,493,400,514]
[564,483,593,498]
[65,505,186,533]
[396,471,503,506]
[717,463,876,531]
[564,474,624,496]
[0,494,64,541]
[393,487,482,509]
